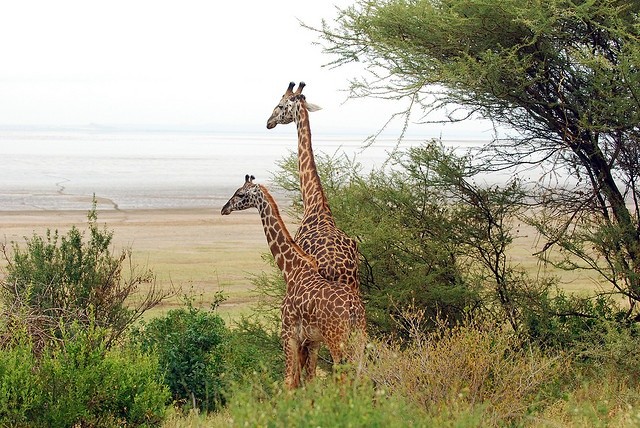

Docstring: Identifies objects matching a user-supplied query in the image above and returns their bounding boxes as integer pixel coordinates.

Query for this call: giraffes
[267,81,359,382]
[220,175,365,397]
